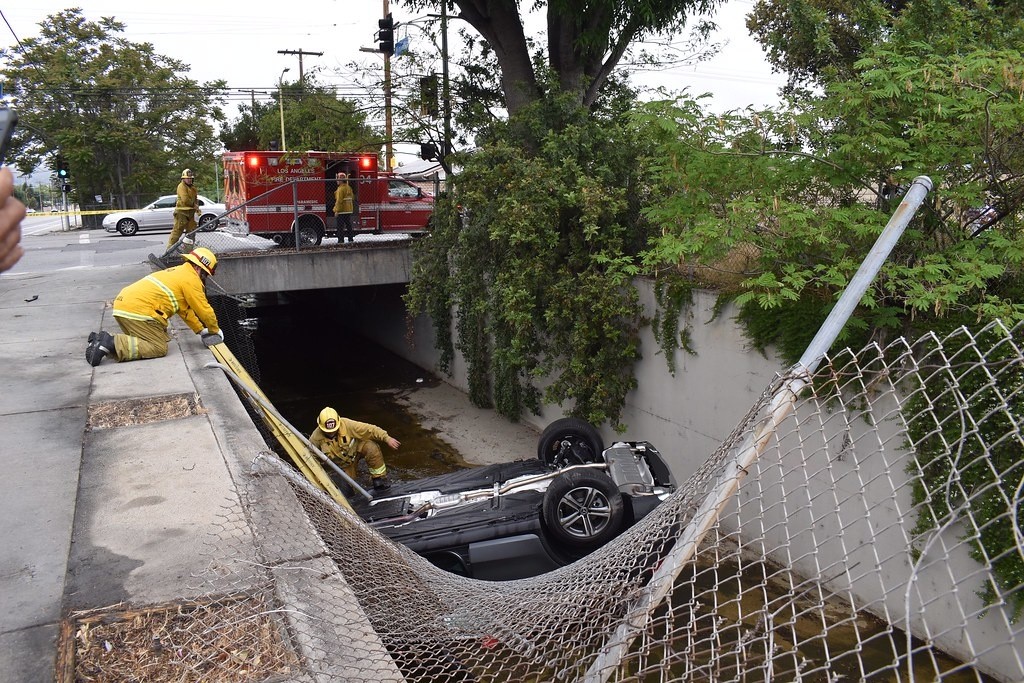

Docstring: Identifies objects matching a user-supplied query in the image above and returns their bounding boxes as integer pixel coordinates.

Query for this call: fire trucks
[222,151,462,251]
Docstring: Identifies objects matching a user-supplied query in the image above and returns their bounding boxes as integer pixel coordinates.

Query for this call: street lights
[279,67,290,152]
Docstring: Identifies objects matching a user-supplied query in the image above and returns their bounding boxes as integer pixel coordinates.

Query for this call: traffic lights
[421,144,434,160]
[379,12,394,58]
[57,155,70,178]
[62,185,70,192]
[420,76,438,121]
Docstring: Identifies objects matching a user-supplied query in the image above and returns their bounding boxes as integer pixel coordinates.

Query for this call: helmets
[317,407,340,433]
[181,169,195,179]
[336,173,346,179]
[180,248,219,277]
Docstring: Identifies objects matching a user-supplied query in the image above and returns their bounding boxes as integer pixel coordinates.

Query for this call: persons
[85,247,225,367]
[165,167,202,254]
[306,407,401,499]
[332,173,355,244]
[0,168,26,274]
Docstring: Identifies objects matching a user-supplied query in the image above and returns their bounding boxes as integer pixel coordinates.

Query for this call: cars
[102,194,227,237]
[347,417,680,643]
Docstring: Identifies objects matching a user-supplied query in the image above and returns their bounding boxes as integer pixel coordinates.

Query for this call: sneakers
[90,331,114,367]
[86,331,99,364]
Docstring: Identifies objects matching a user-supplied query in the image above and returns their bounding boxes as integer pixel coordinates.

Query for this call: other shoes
[373,477,386,490]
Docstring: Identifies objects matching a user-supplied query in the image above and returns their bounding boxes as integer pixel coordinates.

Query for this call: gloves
[216,328,224,341]
[200,328,209,336]
